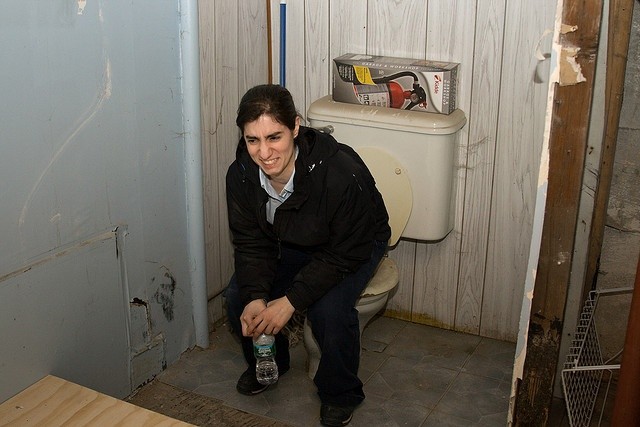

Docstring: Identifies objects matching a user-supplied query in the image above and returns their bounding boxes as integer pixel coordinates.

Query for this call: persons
[221,85,390,426]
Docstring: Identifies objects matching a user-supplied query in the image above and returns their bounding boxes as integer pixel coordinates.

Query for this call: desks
[1,374,197,427]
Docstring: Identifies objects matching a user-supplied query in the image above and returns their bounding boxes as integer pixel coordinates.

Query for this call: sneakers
[236,364,290,395]
[320,402,354,426]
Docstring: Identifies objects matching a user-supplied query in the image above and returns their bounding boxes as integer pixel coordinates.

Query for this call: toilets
[302,94,467,380]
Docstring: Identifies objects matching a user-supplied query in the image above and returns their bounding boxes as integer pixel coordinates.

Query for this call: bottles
[253,331,278,386]
